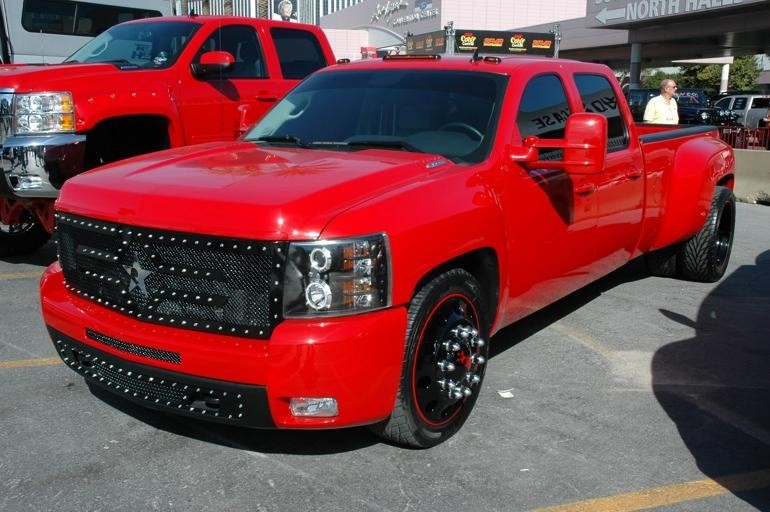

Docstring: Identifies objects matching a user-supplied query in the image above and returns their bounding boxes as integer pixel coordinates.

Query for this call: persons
[643,78,679,125]
[278,0,292,21]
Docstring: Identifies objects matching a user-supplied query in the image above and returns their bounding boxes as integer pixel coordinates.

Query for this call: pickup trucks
[0,16,350,265]
[39,52,736,449]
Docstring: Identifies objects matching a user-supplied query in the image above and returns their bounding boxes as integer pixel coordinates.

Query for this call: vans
[714,95,770,130]
[0,0,175,64]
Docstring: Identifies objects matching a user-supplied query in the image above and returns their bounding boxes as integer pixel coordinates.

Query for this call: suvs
[628,86,738,125]
